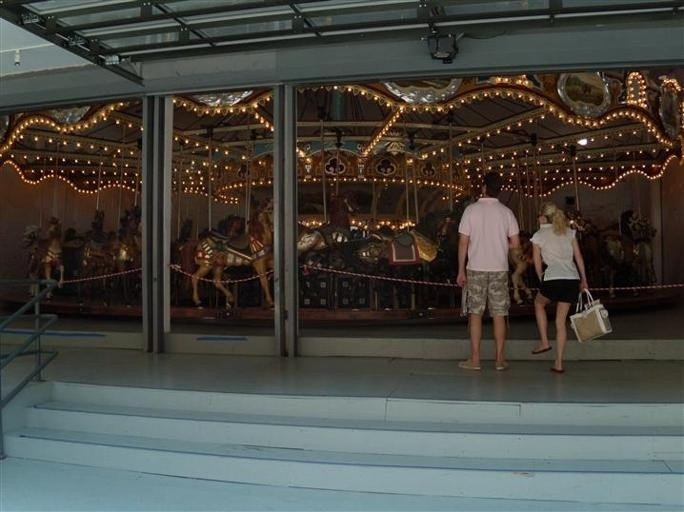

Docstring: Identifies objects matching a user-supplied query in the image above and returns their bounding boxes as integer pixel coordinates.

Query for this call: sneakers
[459,357,481,369]
[495,363,507,371]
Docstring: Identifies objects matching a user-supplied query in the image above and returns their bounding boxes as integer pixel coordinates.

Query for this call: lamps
[178,25,191,44]
[416,0,431,19]
[420,19,458,64]
[46,16,101,57]
[291,15,305,34]
[139,0,154,19]
[549,0,564,17]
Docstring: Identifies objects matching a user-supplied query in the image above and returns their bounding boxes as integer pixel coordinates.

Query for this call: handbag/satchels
[569,303,612,343]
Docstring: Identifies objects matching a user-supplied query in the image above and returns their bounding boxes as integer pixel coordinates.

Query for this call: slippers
[551,367,563,373]
[531,345,552,355]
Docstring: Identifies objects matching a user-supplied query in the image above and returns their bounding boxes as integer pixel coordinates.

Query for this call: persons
[45,217,62,240]
[525,199,591,373]
[446,168,524,372]
